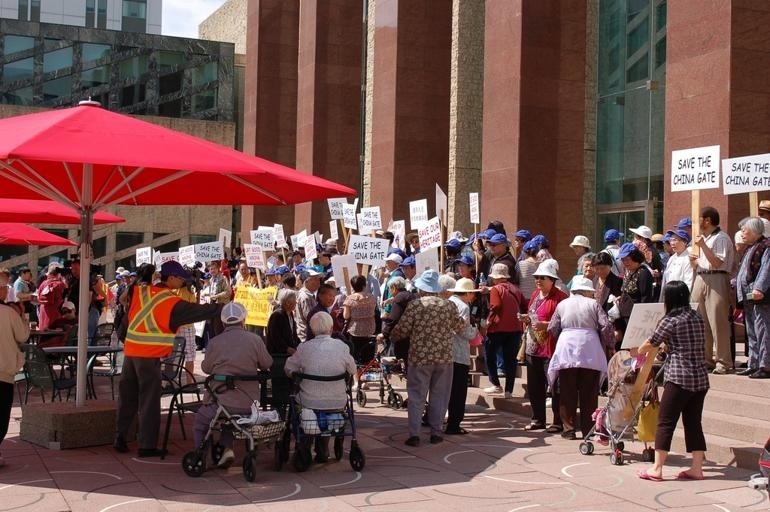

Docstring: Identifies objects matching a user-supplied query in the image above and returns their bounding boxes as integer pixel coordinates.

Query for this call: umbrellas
[0,196,126,226]
[0,99,356,406]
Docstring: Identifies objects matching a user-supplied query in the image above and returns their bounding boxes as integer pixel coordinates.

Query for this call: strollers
[748,432,770,506]
[574,345,660,467]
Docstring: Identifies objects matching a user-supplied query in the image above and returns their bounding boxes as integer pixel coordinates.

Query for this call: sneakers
[216,449,236,471]
[137,448,169,458]
[405,436,419,447]
[294,447,330,465]
[114,444,131,453]
[431,434,443,445]
[444,426,470,436]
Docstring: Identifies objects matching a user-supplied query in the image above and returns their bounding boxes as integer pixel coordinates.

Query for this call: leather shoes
[749,370,769,379]
[736,366,756,375]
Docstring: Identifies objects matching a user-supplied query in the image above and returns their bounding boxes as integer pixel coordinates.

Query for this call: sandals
[525,422,544,429]
[547,424,564,433]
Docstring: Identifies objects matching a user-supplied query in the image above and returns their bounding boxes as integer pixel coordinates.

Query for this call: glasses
[534,276,549,280]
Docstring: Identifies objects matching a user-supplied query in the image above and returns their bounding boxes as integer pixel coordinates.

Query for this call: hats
[265,238,340,288]
[61,301,75,310]
[375,231,419,266]
[221,302,247,324]
[570,275,596,293]
[604,217,693,259]
[569,235,591,249]
[415,256,482,292]
[444,228,546,252]
[488,263,510,279]
[158,260,193,279]
[533,262,558,279]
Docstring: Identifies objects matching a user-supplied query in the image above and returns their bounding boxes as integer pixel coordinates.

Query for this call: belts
[698,270,729,275]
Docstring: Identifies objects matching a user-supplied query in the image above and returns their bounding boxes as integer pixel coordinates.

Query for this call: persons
[0,208,768,481]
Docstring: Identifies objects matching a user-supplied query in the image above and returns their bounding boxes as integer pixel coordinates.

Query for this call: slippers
[674,471,701,480]
[639,471,664,483]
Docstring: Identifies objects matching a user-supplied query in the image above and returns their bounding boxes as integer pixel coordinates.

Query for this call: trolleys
[179,372,286,484]
[279,370,370,477]
[344,336,408,413]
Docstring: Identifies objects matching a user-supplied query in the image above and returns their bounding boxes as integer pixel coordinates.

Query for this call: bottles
[479,272,485,282]
[530,309,538,324]
[110,330,118,347]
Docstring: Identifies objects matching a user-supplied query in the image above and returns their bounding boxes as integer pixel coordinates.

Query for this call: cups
[30,322,37,330]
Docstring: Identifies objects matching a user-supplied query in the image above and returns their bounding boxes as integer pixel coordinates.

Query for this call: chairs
[19,322,207,460]
